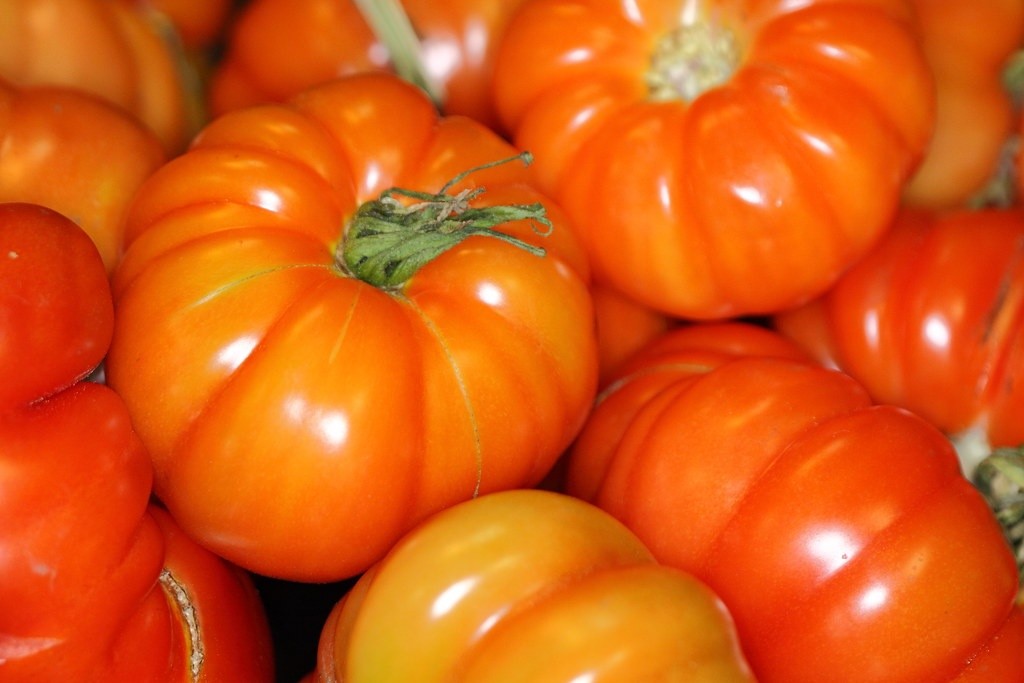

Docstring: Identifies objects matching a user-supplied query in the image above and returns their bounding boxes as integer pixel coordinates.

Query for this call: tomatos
[1,1,1024,683]
